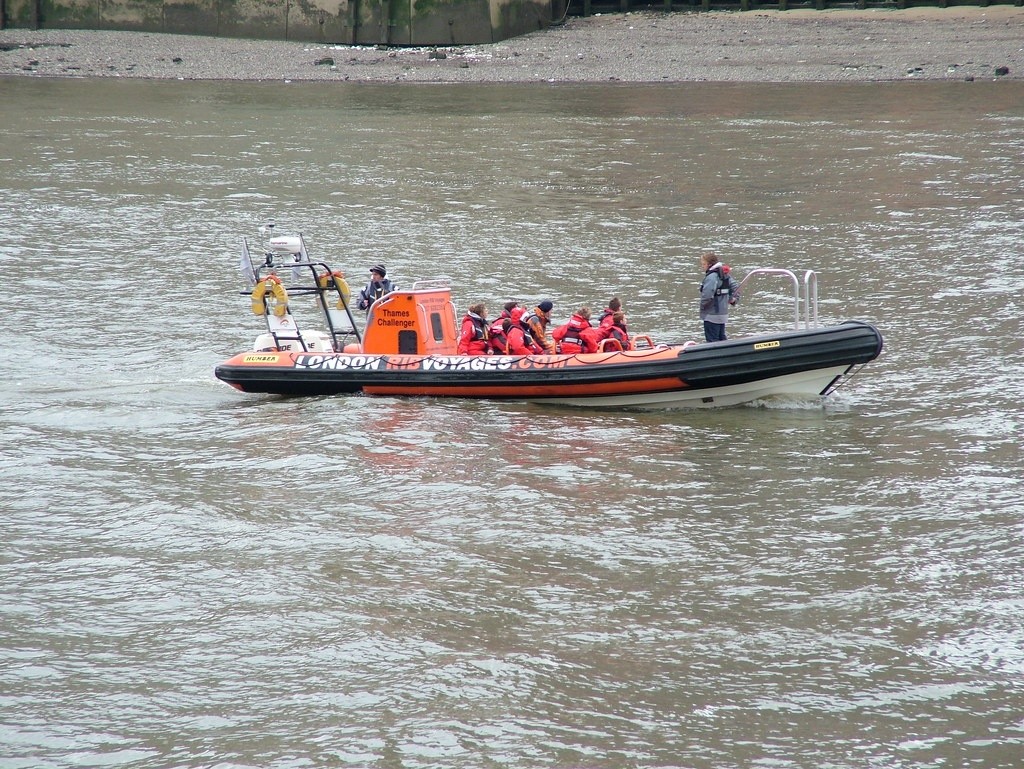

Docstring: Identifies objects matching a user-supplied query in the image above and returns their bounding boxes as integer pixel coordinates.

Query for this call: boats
[214,233,887,414]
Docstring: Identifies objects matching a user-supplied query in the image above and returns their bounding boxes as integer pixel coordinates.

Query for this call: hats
[370,264,386,278]
[539,301,553,312]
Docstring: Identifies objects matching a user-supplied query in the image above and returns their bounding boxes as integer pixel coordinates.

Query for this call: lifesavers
[315,275,351,311]
[251,279,290,317]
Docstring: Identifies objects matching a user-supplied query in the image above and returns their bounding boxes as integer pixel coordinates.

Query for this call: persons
[457,300,556,355]
[700,251,740,342]
[595,297,637,351]
[356,264,400,321]
[553,307,598,354]
